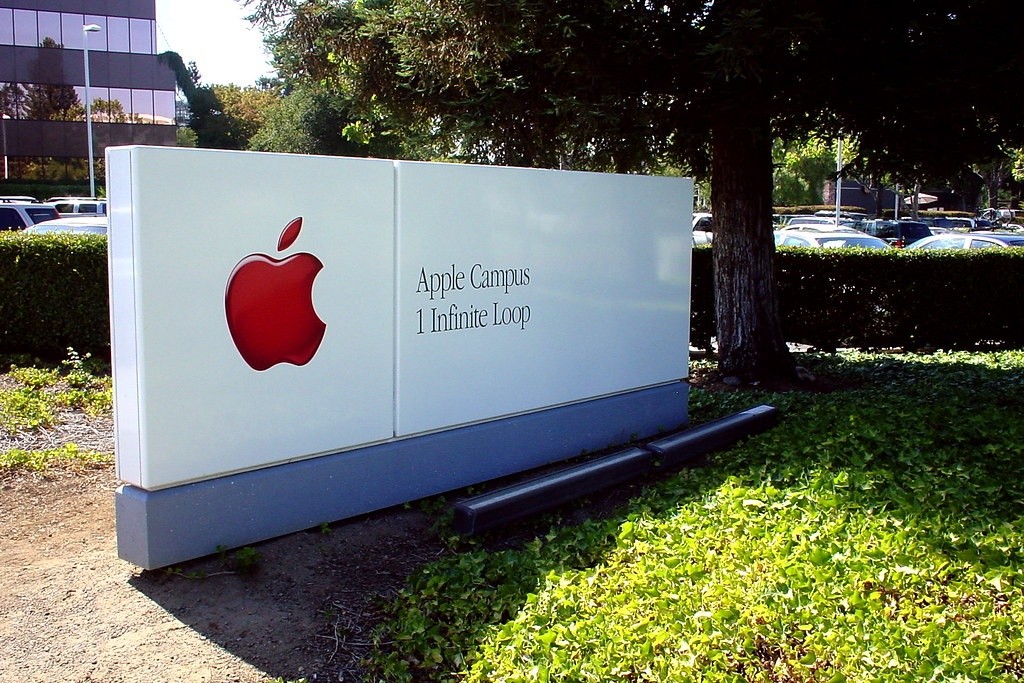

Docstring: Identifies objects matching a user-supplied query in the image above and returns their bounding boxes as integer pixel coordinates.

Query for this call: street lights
[83,23,101,198]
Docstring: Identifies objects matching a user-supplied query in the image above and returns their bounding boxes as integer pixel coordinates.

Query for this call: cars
[773,208,1024,233]
[774,224,893,252]
[902,234,1024,253]
[692,213,714,247]
[0,196,109,241]
